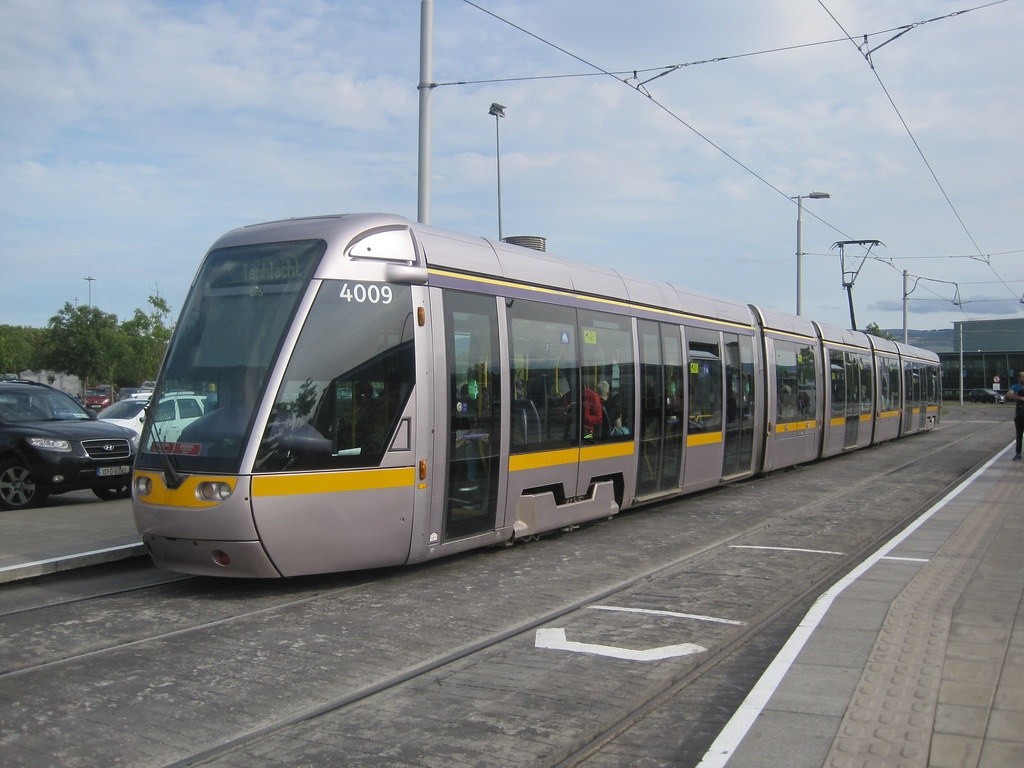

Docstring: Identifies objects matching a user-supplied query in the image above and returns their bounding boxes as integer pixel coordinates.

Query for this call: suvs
[0,377,142,509]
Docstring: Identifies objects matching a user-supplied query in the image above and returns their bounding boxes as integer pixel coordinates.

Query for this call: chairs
[569,400,616,443]
[493,398,545,448]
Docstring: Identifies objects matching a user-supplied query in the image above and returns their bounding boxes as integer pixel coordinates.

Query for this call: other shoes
[1012,454,1022,461]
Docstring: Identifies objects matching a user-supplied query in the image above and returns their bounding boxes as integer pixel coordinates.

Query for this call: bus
[129,214,947,586]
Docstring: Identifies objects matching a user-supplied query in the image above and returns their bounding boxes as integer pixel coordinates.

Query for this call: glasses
[349,392,367,401]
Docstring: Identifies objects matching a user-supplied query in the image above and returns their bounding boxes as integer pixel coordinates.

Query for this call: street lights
[792,191,829,316]
[488,102,508,242]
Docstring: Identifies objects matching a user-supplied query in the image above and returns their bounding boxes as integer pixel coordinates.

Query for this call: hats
[782,385,791,396]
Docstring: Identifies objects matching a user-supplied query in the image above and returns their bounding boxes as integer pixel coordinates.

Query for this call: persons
[335,358,815,497]
[1006,372,1024,460]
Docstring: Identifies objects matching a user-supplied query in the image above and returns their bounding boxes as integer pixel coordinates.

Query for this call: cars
[78,381,206,451]
[971,388,1006,403]
